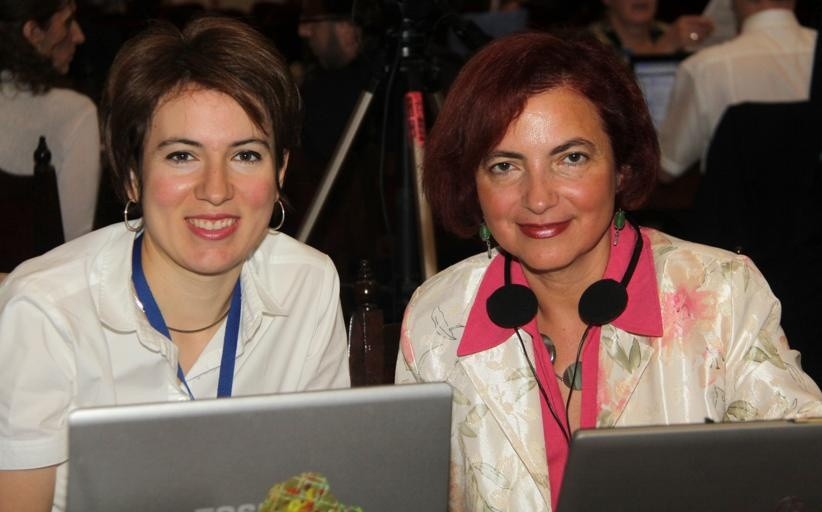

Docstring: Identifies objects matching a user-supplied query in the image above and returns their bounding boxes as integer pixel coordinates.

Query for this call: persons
[585,1,712,55]
[1,19,350,512]
[0,0,99,274]
[477,1,529,43]
[656,1,818,186]
[395,33,822,512]
[297,2,399,159]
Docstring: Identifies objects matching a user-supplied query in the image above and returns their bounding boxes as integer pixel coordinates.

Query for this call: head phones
[482,233,646,329]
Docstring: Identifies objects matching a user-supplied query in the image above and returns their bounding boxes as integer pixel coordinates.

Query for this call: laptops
[67,381,453,512]
[557,417,822,512]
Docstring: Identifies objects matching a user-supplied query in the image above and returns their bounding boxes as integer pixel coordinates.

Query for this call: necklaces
[166,306,229,334]
[539,332,581,392]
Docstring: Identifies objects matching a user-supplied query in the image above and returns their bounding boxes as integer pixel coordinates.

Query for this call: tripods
[289,89,495,278]
